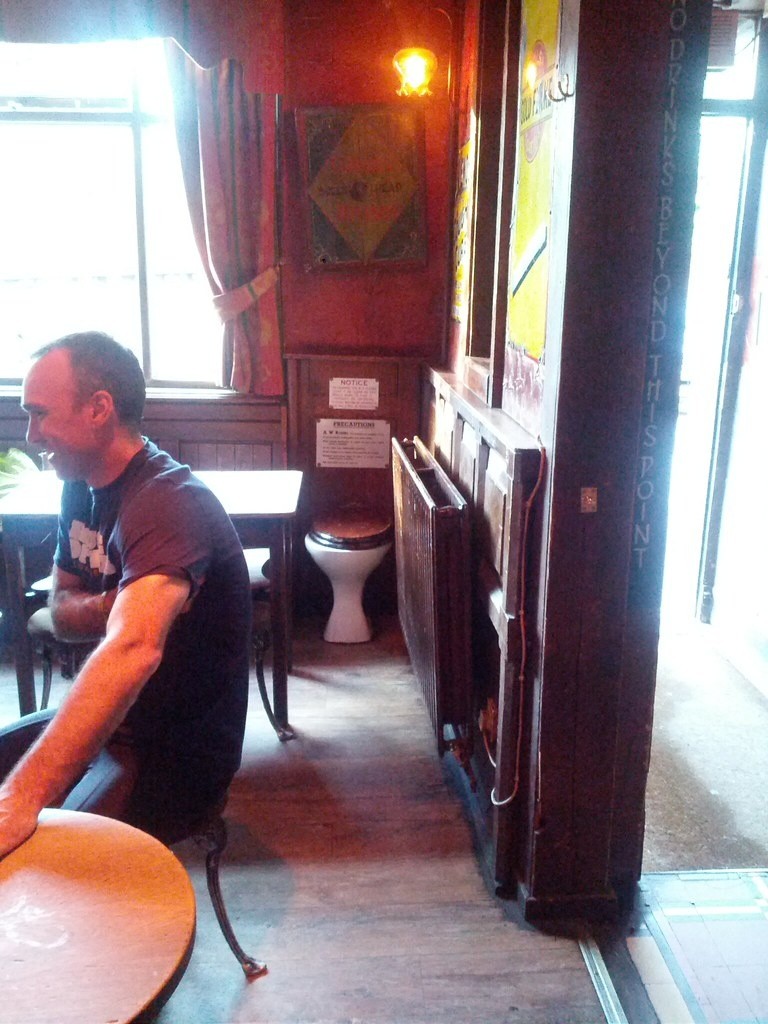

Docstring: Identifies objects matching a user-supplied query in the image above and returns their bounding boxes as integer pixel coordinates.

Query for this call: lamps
[391,3,472,117]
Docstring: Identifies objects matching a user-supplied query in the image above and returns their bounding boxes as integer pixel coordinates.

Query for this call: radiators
[391,433,481,794]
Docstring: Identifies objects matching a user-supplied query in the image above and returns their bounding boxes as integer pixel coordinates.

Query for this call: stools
[21,548,298,978]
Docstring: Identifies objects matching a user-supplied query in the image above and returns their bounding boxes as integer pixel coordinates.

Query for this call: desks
[0,805,197,1024]
[0,469,304,742]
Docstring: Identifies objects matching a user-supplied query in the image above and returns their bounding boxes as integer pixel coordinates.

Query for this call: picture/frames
[293,97,430,274]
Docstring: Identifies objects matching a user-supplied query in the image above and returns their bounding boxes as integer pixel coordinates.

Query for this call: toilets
[304,508,394,644]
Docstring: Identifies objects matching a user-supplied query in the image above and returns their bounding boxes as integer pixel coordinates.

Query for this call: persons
[1,331,251,861]
